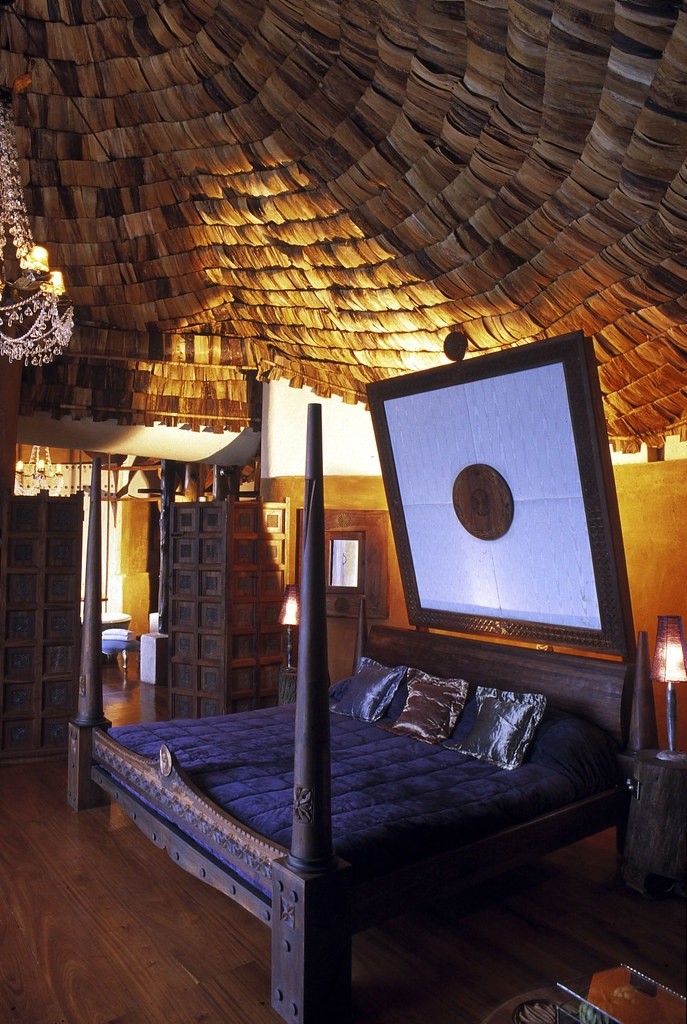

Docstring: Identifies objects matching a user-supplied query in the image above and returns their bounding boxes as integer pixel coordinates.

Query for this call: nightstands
[624,749,687,898]
[278,671,330,706]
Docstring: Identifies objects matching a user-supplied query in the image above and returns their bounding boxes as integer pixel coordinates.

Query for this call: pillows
[444,686,547,771]
[329,657,408,722]
[376,668,470,744]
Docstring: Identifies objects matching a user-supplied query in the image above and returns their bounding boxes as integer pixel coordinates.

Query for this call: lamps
[649,615,687,763]
[277,584,301,674]
[5,245,66,295]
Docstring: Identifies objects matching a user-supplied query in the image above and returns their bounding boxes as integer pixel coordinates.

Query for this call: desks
[80,613,133,664]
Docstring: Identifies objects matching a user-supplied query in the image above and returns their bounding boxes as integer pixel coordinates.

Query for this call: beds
[63,401,661,1024]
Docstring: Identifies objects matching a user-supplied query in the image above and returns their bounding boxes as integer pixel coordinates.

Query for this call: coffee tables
[555,962,687,1024]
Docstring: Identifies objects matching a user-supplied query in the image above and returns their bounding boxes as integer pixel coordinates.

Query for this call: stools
[101,640,140,668]
